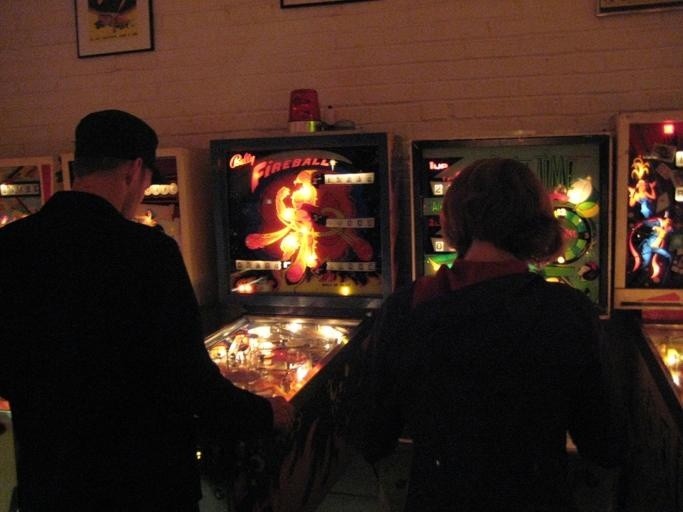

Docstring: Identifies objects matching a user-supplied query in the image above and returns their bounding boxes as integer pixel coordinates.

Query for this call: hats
[72,110,171,186]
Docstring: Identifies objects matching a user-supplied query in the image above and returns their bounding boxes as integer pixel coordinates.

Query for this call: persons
[0,110,297,512]
[360,157,605,512]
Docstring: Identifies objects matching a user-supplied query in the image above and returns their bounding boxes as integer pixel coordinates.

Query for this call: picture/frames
[73,0,155,59]
